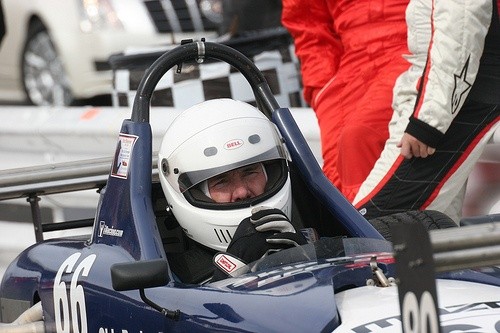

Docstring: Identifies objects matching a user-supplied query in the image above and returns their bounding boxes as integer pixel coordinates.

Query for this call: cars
[0,0,236,108]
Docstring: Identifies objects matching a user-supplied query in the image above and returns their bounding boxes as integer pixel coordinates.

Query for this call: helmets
[157,98,292,252]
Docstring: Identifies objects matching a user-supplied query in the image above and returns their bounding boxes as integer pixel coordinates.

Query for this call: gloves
[214,215,287,281]
[250,205,316,261]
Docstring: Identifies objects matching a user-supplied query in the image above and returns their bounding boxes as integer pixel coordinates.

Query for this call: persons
[351,0,500,221]
[281,0,411,204]
[156,98,348,286]
